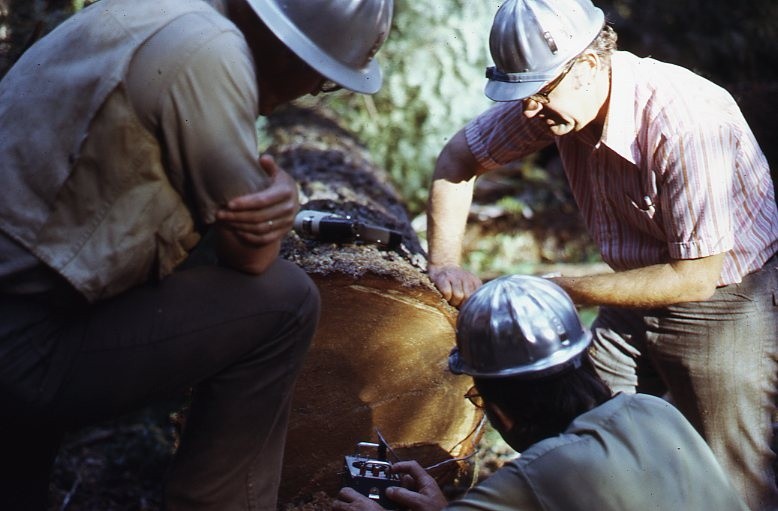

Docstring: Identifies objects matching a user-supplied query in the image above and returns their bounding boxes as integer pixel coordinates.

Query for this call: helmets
[246,0,393,95]
[448,274,594,376]
[485,0,605,102]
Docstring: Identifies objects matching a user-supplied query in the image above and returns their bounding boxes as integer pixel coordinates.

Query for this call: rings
[268,221,272,231]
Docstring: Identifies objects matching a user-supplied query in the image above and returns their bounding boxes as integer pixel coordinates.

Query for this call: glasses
[527,57,579,104]
[319,79,342,92]
[464,384,513,408]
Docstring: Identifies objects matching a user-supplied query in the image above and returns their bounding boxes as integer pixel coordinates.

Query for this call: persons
[325,274,751,511]
[0,0,393,511]
[427,0,778,511]
